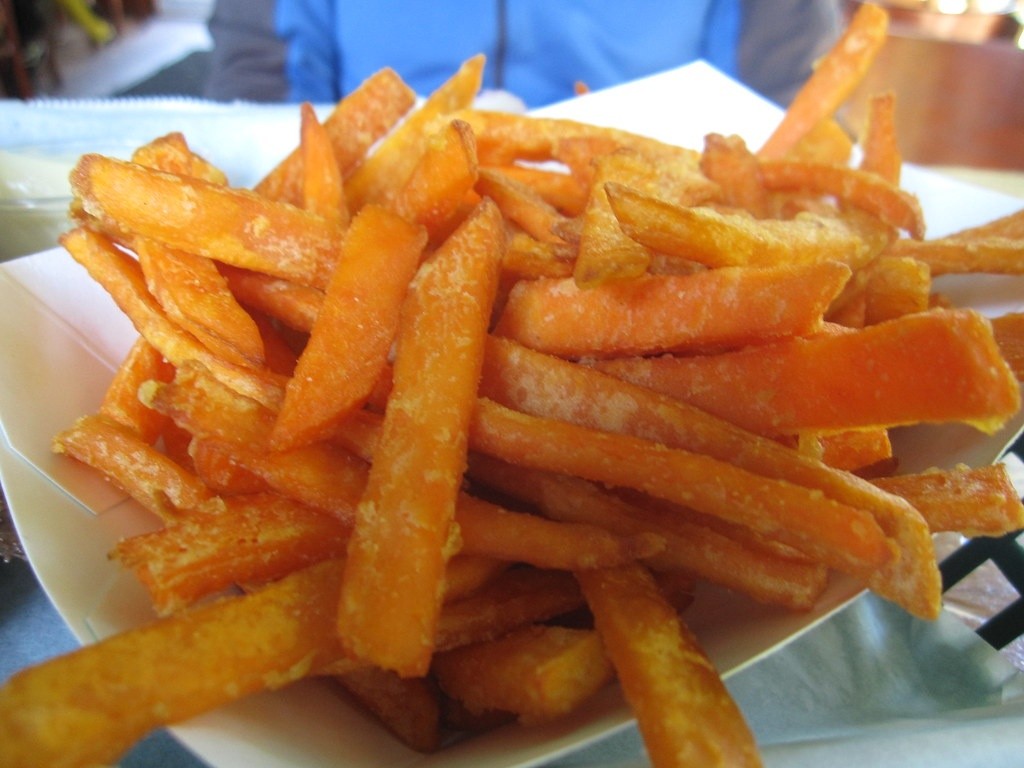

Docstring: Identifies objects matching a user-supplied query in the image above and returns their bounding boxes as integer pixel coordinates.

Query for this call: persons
[209,0,843,112]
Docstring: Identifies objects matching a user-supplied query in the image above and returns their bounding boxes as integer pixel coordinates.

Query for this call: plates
[0,60,1024,768]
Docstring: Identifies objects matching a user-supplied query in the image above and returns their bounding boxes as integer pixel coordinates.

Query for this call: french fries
[1,1,1024,768]
